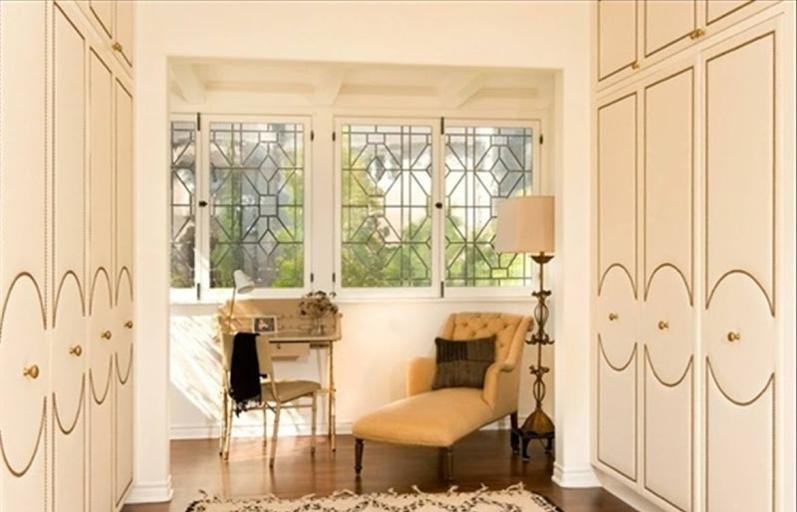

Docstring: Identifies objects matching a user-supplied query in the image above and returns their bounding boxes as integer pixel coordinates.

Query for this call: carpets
[184,483,563,512]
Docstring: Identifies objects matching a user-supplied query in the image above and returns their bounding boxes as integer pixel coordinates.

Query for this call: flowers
[297,291,340,319]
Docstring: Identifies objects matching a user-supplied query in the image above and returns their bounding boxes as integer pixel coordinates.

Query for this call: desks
[218,297,343,451]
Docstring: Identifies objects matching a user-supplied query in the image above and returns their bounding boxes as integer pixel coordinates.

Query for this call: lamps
[493,195,555,457]
[227,269,255,331]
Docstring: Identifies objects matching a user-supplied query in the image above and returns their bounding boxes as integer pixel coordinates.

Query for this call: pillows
[432,333,497,389]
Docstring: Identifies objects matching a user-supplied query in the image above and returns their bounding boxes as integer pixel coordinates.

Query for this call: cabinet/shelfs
[590,1,797,512]
[0,0,136,512]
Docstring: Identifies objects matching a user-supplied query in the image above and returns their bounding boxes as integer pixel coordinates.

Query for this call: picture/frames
[252,315,277,332]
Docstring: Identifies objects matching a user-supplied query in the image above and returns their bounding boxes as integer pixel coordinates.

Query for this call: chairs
[223,332,322,469]
[353,312,532,482]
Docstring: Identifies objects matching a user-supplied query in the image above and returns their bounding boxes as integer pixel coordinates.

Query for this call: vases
[309,318,323,336]
